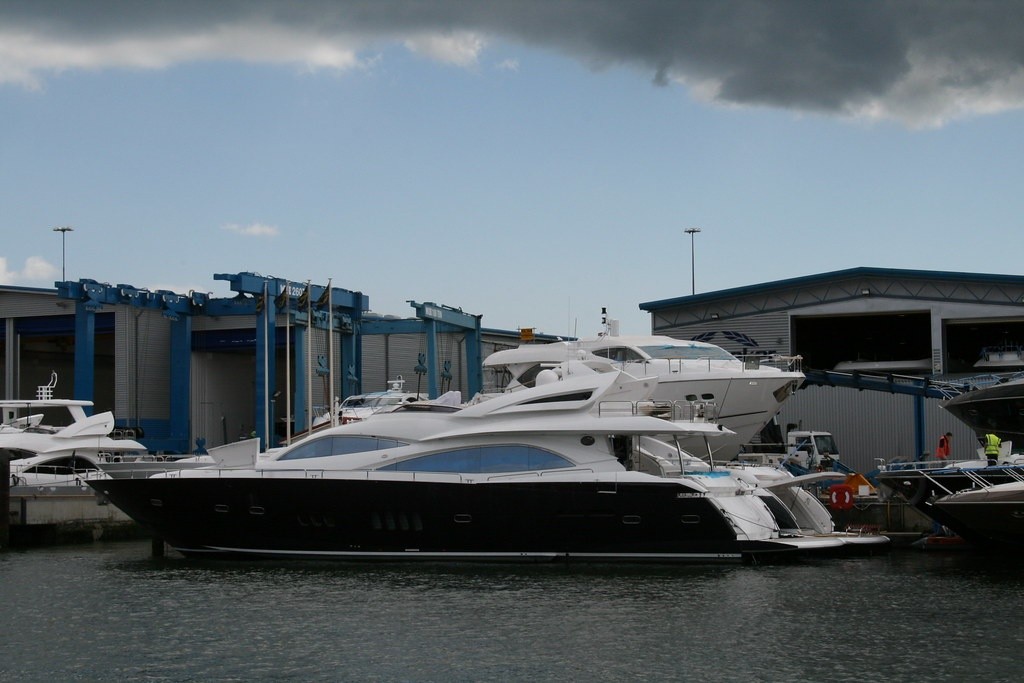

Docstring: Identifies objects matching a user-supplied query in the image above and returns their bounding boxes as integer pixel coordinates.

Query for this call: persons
[982,428,1002,466]
[937,432,952,467]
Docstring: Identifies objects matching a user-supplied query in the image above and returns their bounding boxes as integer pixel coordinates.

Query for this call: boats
[0,305,1023,566]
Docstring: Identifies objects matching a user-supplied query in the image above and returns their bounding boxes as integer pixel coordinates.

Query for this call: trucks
[738,429,842,470]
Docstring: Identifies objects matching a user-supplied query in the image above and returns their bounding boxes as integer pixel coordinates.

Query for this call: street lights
[53,227,74,283]
[683,226,703,297]
